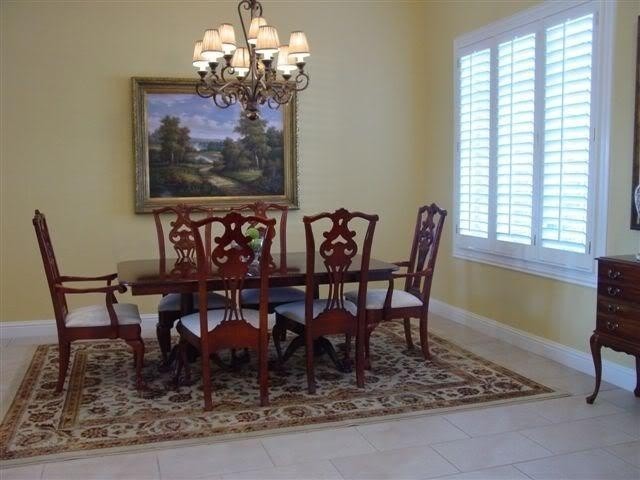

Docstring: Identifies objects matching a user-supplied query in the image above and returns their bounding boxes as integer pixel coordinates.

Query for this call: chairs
[32,208,144,391]
[165,212,280,411]
[150,202,238,374]
[344,203,447,369]
[272,208,379,395]
[224,197,306,352]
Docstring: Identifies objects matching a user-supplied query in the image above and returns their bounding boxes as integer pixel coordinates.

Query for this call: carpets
[1,318,574,478]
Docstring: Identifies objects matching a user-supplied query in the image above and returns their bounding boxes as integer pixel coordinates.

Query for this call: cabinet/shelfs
[586,254,640,408]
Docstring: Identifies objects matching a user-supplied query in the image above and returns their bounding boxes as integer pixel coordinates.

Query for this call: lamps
[192,0,311,121]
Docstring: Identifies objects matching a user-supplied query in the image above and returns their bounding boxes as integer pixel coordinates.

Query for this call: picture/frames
[129,74,301,215]
